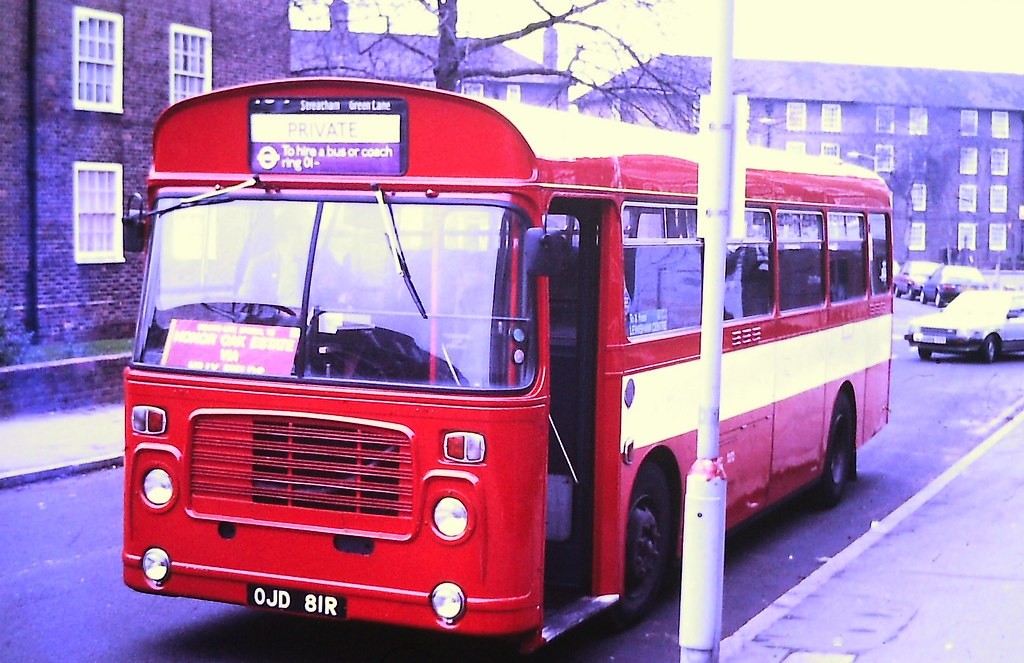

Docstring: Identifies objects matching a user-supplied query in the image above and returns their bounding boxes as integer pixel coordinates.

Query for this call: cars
[904,290,1024,363]
[919,265,988,308]
[894,260,943,299]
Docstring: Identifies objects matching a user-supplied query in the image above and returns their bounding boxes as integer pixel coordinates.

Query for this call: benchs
[550,247,884,348]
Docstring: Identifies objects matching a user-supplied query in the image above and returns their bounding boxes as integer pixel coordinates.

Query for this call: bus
[121,78,894,659]
